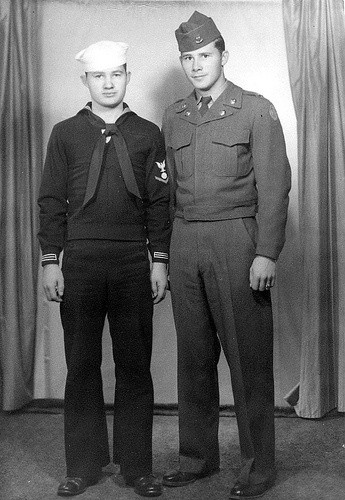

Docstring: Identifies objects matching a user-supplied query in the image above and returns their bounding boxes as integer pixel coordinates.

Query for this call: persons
[160,8,291,499]
[34,41,174,496]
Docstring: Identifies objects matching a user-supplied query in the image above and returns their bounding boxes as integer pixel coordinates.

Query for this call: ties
[198,97,213,117]
[80,111,142,209]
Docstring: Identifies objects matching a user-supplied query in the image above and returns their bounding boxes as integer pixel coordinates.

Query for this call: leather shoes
[164,464,220,486]
[230,471,277,499]
[121,471,164,497]
[58,469,105,497]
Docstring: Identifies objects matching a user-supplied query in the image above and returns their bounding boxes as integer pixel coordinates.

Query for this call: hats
[75,41,129,73]
[173,10,220,51]
